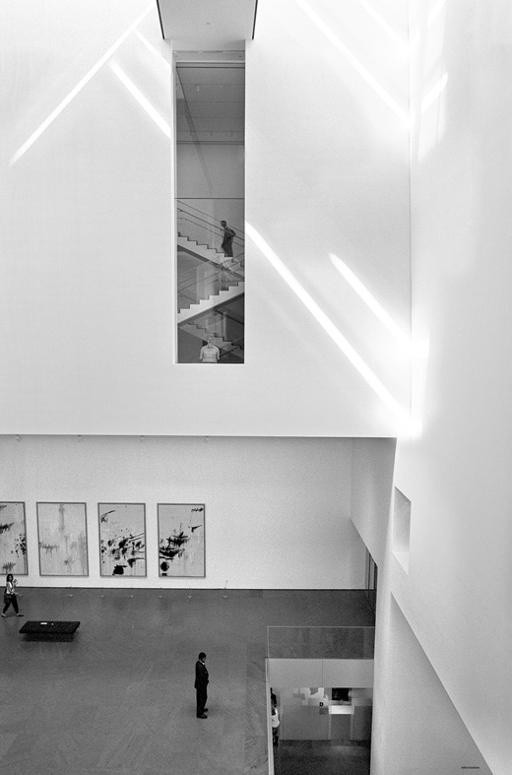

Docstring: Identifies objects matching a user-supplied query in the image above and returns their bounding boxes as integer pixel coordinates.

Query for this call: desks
[19,621,80,642]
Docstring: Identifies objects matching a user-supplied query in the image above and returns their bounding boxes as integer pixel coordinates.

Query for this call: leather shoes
[195,708,208,719]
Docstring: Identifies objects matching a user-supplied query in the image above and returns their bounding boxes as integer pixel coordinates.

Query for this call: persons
[194,652,209,719]
[1,574,24,617]
[221,220,236,257]
[200,335,220,363]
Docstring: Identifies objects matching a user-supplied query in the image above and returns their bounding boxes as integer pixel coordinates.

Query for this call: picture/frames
[0,501,207,578]
